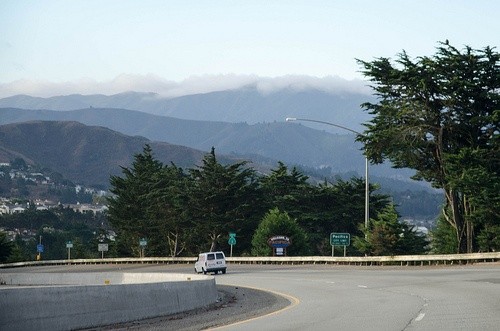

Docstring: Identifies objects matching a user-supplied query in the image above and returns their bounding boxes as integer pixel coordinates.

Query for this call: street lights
[285,117,371,255]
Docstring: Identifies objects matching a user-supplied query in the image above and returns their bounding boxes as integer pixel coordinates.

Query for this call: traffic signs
[66,240,73,248]
[228,232,236,245]
[330,233,350,246]
[139,237,147,246]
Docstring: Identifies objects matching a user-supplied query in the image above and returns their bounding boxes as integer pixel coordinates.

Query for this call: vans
[194,252,227,274]
